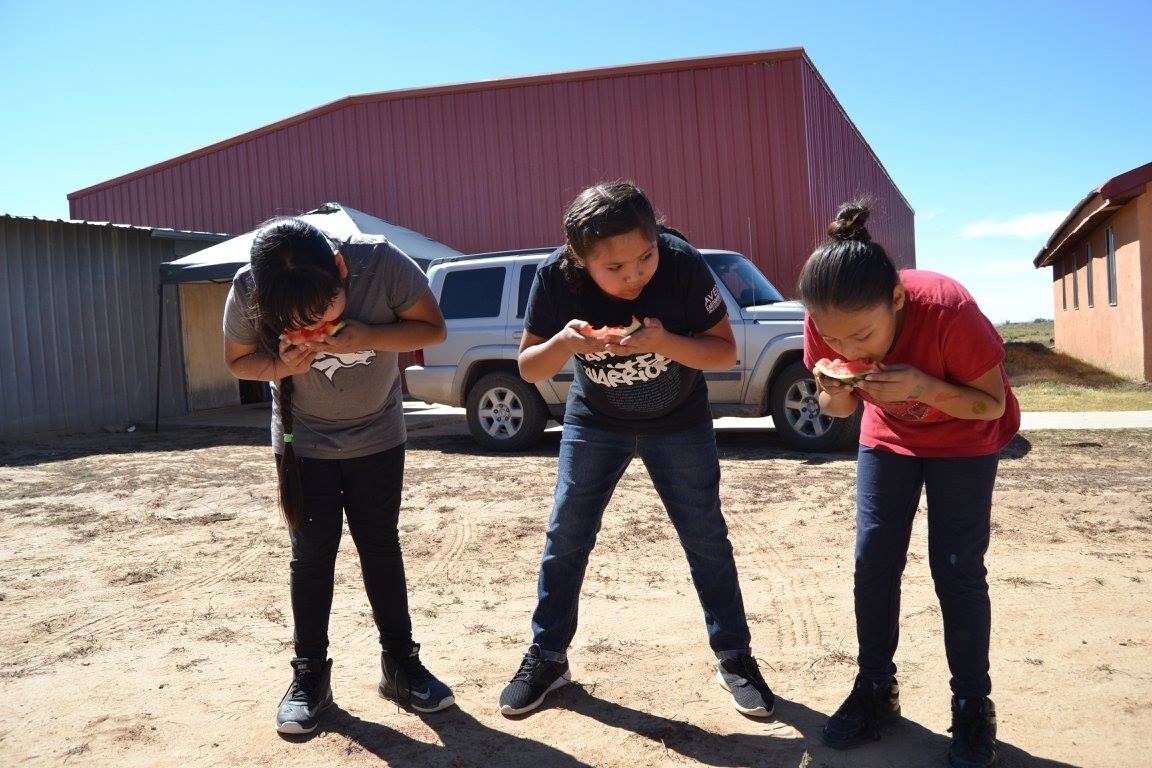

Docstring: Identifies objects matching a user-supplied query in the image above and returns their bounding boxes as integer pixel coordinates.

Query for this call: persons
[497,179,777,719]
[224,216,454,734]
[794,197,1021,768]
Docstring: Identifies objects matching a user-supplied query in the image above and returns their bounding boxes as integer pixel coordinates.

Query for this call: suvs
[404,245,864,453]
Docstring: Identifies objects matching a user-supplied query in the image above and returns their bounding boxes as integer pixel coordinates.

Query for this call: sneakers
[379,640,455,712]
[275,657,334,732]
[948,697,997,768]
[716,651,775,716]
[822,675,901,749]
[499,643,571,715]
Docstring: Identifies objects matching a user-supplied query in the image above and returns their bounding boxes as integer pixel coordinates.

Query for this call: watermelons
[815,357,881,379]
[279,321,347,346]
